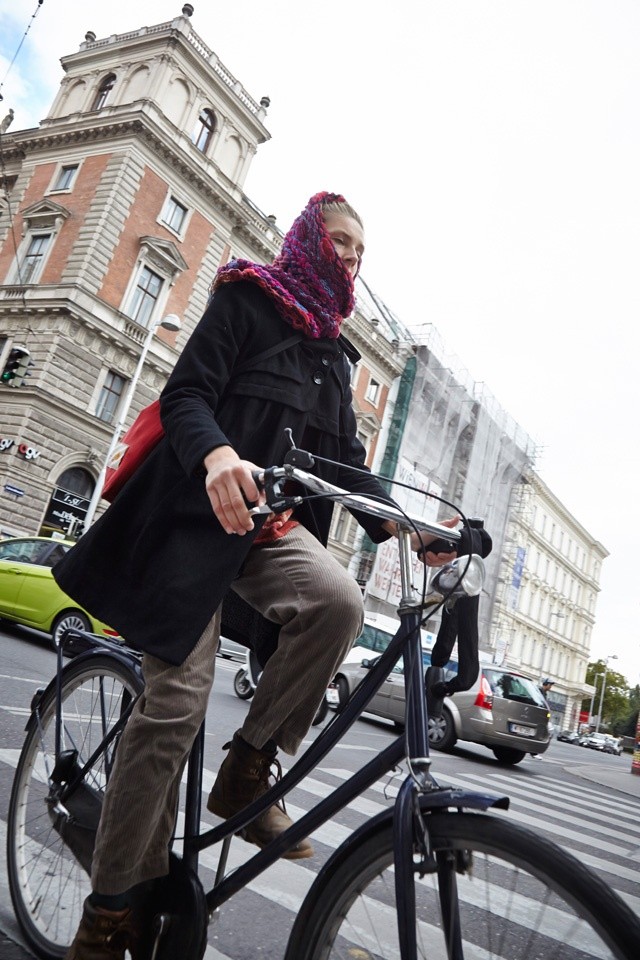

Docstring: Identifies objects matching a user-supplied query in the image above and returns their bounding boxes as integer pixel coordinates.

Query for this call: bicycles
[6,425,640,960]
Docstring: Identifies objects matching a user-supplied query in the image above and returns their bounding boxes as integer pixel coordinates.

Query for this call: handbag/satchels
[101,399,165,504]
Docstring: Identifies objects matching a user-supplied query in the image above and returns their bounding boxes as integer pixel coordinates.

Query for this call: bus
[350,609,496,691]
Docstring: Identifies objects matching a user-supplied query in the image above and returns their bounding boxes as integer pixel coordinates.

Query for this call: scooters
[233,663,341,727]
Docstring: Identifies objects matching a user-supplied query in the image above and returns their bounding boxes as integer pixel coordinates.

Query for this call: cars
[0,529,132,660]
[555,728,624,757]
[326,646,552,767]
[217,635,250,661]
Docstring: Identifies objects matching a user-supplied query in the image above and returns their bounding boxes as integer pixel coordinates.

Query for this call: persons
[69,193,463,960]
[530,678,553,759]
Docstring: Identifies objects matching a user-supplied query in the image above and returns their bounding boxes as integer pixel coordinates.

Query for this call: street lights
[586,672,606,724]
[75,311,184,541]
[595,654,618,733]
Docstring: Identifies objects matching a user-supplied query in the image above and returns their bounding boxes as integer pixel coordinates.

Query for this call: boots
[207,731,314,859]
[65,895,140,960]
